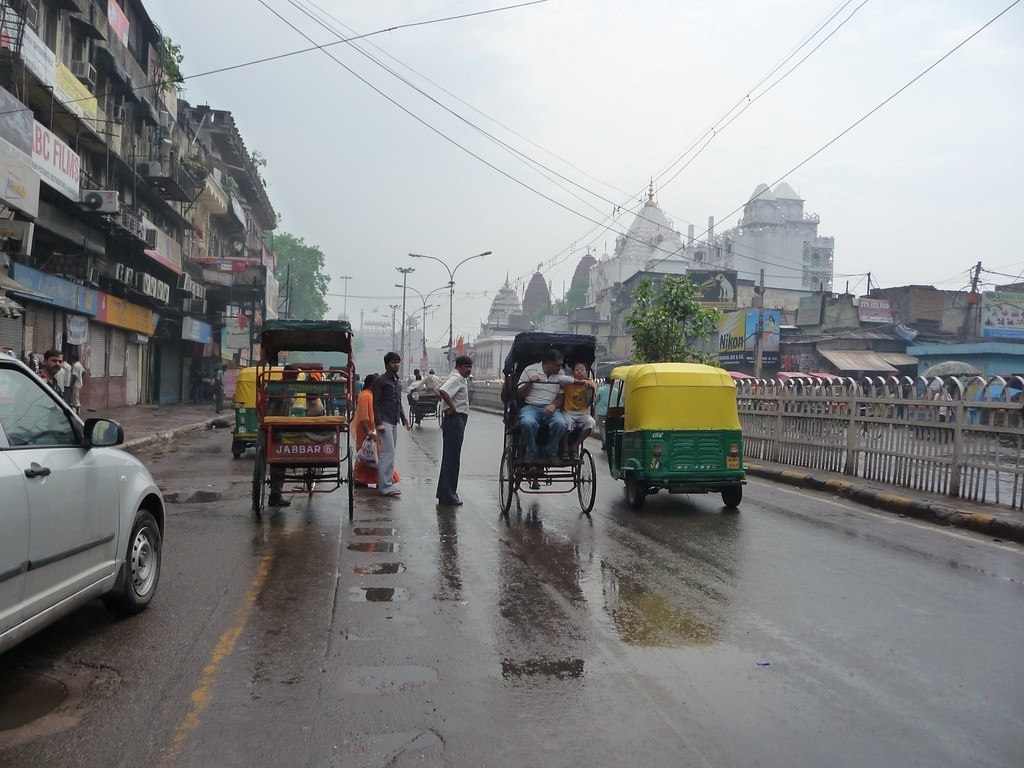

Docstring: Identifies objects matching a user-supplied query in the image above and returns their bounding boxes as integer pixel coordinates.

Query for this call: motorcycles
[604,363,748,507]
[230,365,326,459]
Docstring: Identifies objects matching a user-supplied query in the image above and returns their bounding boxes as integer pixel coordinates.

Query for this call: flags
[456,337,464,355]
[236,314,249,330]
[253,311,262,327]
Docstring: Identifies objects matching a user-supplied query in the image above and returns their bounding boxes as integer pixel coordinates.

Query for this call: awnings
[819,348,899,372]
[876,349,920,365]
[728,371,847,384]
[0,270,54,301]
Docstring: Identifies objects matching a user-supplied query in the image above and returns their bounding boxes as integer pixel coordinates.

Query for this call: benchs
[512,368,594,465]
[260,415,349,457]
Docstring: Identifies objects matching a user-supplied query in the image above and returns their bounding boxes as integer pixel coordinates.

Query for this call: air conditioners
[134,271,169,303]
[75,61,97,88]
[183,298,207,314]
[81,189,119,214]
[114,104,126,124]
[177,271,190,291]
[138,162,159,176]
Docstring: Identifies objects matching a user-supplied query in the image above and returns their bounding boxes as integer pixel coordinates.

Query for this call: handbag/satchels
[356,436,378,468]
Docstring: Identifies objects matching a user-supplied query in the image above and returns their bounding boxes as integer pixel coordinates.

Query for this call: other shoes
[523,453,536,465]
[560,449,569,460]
[601,441,607,450]
[569,451,580,460]
[546,455,560,466]
[354,480,368,488]
[380,488,401,496]
[268,493,291,506]
[439,497,463,505]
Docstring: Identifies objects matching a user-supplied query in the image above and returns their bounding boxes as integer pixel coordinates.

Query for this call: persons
[70,353,86,415]
[36,349,63,399]
[350,374,400,490]
[268,364,300,507]
[764,315,776,342]
[306,367,442,431]
[218,364,228,411]
[435,356,473,505]
[214,378,223,414]
[55,366,65,398]
[933,386,953,445]
[517,349,598,466]
[373,352,411,496]
[501,369,542,490]
[593,376,621,449]
[61,355,72,406]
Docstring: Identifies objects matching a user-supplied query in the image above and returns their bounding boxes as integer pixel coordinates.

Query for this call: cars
[1,351,164,657]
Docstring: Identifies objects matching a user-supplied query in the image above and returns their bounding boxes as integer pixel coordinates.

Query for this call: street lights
[339,275,355,321]
[387,304,433,374]
[396,284,452,377]
[408,251,493,375]
[396,267,416,383]
[376,314,421,378]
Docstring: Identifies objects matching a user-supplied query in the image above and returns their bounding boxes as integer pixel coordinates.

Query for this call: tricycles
[497,332,597,514]
[407,387,443,427]
[250,318,356,522]
